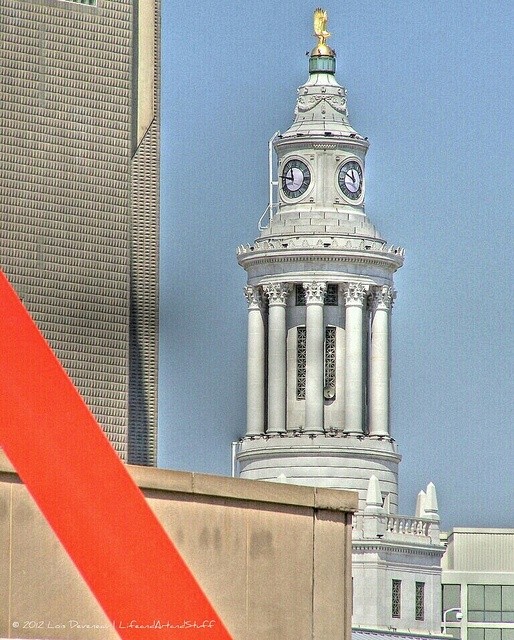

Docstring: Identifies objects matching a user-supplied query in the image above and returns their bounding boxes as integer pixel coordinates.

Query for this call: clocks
[278,154,316,205]
[334,155,365,205]
